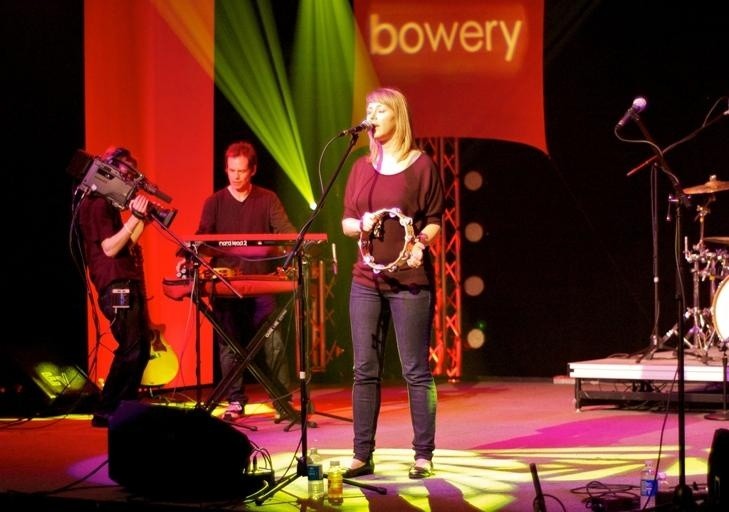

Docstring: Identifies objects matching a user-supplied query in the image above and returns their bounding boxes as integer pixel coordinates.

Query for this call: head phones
[105,148,129,166]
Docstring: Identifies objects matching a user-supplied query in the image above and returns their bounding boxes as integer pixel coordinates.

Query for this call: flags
[346,1,552,157]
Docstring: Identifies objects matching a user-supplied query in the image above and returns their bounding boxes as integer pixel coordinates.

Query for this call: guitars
[140,319,178,385]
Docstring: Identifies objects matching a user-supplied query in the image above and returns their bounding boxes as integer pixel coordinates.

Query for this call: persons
[73,143,163,429]
[337,86,443,480]
[196,138,298,427]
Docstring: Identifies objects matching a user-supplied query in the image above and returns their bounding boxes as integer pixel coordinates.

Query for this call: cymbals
[683,181,729,194]
[704,237,729,243]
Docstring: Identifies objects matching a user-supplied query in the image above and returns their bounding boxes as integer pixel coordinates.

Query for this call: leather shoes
[324,459,374,478]
[408,459,434,479]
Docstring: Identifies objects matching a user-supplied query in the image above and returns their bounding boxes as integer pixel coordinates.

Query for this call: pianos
[163,233,327,298]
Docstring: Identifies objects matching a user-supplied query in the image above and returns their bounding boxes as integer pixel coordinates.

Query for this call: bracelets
[123,224,132,235]
[360,215,365,233]
[416,231,430,248]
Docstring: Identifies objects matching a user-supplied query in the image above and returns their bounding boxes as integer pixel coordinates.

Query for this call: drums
[711,274,729,349]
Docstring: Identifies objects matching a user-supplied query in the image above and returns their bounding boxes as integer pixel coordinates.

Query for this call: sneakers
[224,400,243,419]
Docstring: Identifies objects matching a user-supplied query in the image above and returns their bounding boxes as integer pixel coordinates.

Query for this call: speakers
[107,400,254,499]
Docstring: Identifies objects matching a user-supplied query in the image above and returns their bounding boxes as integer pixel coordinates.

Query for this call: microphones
[616,96,647,128]
[337,119,373,137]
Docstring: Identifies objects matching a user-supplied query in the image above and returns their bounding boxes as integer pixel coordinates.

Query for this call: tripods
[274,261,353,432]
[626,115,721,364]
[255,132,390,506]
[151,213,258,431]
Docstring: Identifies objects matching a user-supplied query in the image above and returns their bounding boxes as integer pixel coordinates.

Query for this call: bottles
[328,460,343,499]
[640,461,669,509]
[306,448,324,500]
